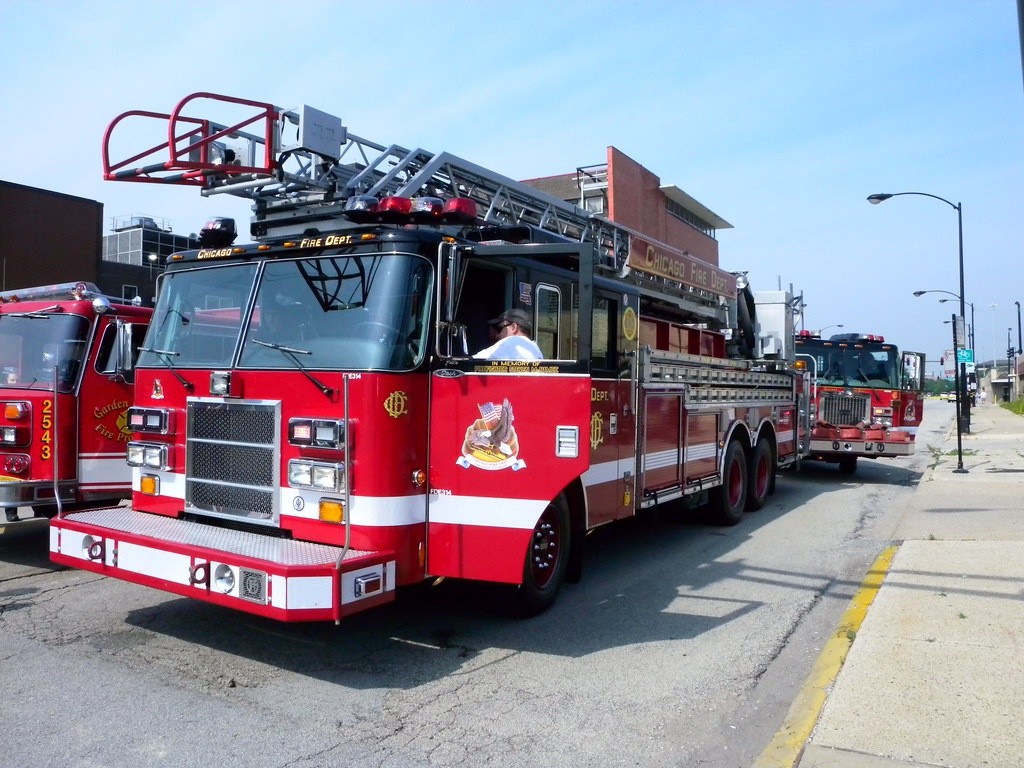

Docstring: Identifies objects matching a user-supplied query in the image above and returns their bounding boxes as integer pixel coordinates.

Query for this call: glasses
[497,324,511,333]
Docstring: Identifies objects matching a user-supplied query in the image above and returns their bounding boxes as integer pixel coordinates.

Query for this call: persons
[473,307,544,360]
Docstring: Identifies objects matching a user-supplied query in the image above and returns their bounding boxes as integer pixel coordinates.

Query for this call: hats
[488,308,532,331]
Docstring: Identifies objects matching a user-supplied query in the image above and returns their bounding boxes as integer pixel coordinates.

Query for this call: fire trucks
[47,92,817,627]
[793,327,927,477]
[0,283,262,544]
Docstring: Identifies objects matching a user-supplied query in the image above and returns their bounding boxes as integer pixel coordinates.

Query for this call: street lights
[939,299,977,408]
[868,192,969,474]
[818,323,845,339]
[942,320,972,350]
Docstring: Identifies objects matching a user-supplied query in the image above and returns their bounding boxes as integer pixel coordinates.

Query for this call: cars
[948,390,958,402]
[938,392,949,400]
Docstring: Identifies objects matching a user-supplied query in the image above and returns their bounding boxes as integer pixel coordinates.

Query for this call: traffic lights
[1006,347,1015,358]
